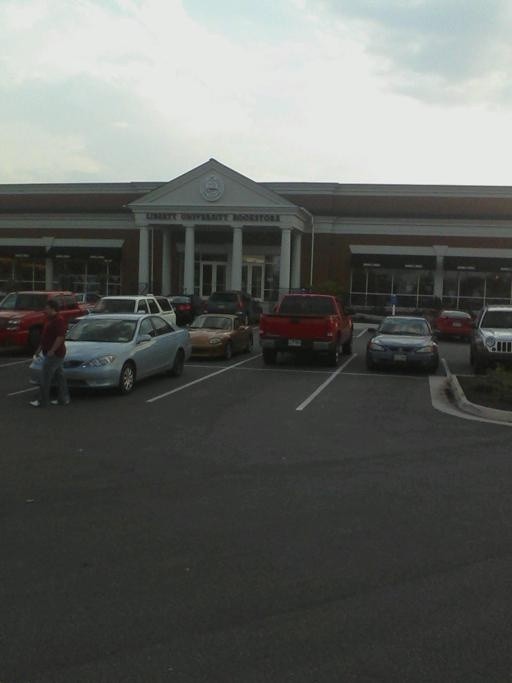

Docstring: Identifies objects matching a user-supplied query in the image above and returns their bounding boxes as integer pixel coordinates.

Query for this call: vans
[207,291,264,325]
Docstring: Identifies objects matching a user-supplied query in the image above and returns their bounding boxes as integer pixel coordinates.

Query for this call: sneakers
[29,399,73,407]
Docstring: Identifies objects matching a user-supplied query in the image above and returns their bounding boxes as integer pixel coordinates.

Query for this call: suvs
[474,303,512,361]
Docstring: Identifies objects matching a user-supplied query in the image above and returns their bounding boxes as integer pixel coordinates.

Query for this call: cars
[182,313,256,360]
[433,308,474,341]
[28,313,193,396]
[364,315,440,373]
[0,289,206,349]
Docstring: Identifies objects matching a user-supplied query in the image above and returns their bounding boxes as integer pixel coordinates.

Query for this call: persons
[30,300,70,407]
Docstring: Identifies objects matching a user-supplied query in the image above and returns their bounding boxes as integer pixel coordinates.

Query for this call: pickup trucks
[258,288,357,366]
